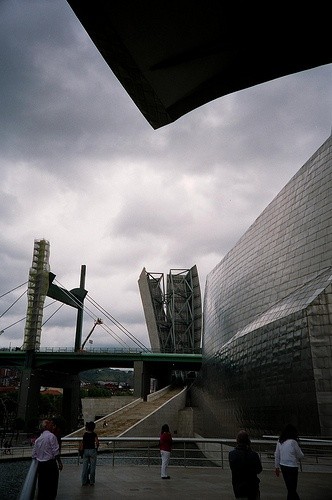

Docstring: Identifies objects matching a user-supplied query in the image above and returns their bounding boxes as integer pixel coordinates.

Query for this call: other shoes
[162,475,170,479]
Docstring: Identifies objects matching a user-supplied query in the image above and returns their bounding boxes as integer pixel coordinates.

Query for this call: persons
[81,421,99,486]
[274,424,304,500]
[32,419,63,500]
[229,432,262,500]
[159,424,173,479]
